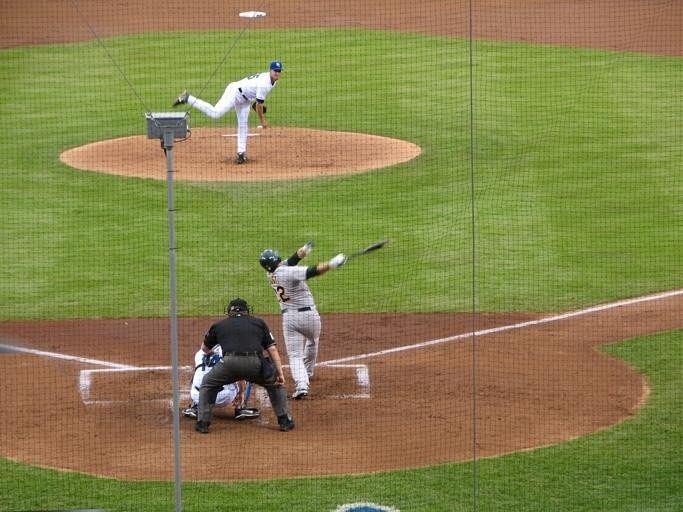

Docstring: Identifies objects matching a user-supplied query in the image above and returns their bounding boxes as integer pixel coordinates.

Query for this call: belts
[223,352,263,357]
[193,385,224,394]
[238,84,251,103]
[281,305,318,314]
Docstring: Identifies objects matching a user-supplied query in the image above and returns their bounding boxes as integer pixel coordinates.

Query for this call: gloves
[329,252,348,269]
[302,239,316,257]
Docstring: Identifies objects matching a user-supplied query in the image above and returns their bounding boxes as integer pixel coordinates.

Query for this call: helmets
[258,248,281,272]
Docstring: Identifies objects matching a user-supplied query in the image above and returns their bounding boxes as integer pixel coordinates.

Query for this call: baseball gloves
[205,352,220,367]
[253,102,268,115]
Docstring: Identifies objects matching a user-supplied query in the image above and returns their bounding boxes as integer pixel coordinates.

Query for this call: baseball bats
[345,242,388,258]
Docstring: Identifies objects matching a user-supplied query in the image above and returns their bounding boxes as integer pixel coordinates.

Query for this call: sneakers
[172,89,189,108]
[181,406,198,418]
[276,413,295,432]
[235,152,247,163]
[193,420,211,434]
[234,407,261,420]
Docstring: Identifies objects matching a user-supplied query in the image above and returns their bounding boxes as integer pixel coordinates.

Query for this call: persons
[193,298,294,433]
[258,242,347,402]
[179,345,260,423]
[171,60,282,165]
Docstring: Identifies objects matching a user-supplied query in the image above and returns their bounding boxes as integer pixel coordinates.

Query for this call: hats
[227,296,250,312]
[269,60,284,72]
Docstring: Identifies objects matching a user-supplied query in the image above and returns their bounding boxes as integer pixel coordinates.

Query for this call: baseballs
[257,125,262,130]
